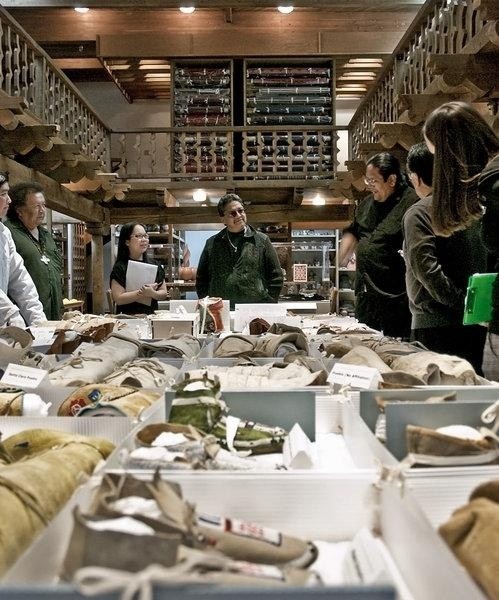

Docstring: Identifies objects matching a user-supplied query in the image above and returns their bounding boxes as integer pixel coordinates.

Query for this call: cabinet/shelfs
[257,225,357,315]
[109,224,196,308]
[167,56,338,180]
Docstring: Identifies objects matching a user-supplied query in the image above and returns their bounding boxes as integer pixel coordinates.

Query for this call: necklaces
[227,228,237,252]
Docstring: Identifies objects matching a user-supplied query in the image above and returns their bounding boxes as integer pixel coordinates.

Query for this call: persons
[195,194,283,311]
[339,153,421,342]
[110,221,167,315]
[0,175,63,331]
[401,101,499,381]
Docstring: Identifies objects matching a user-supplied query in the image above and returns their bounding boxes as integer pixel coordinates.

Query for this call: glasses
[32,203,45,208]
[227,209,244,217]
[131,233,149,239]
[364,177,382,185]
